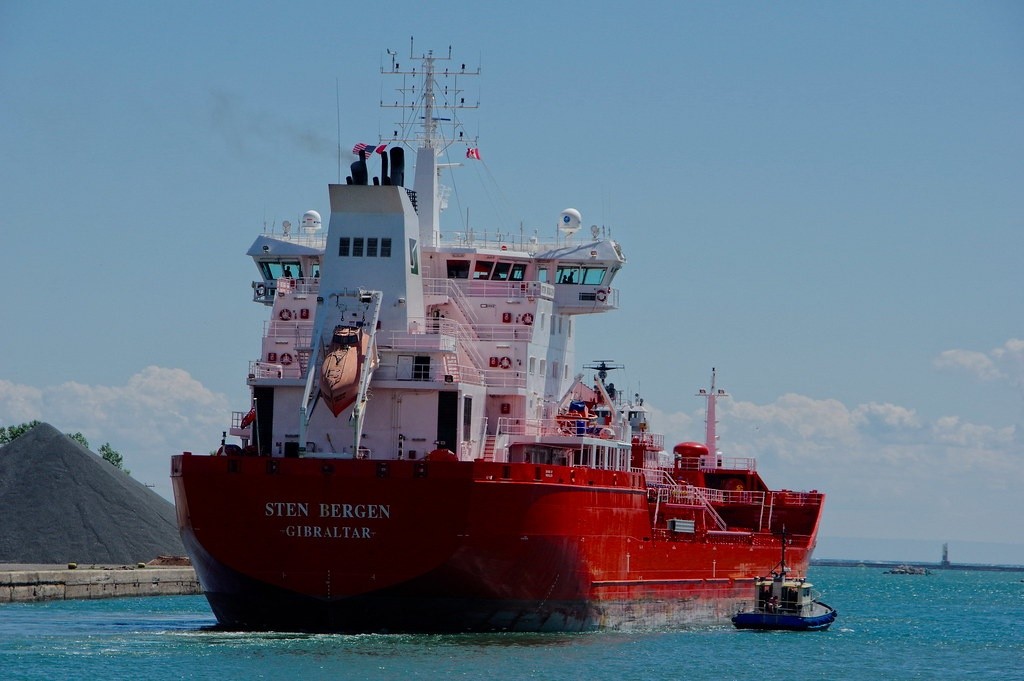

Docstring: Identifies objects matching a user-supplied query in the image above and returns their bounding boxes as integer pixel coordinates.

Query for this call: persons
[568,272,574,284]
[607,383,618,400]
[314,270,319,283]
[562,274,567,283]
[282,266,294,279]
[769,596,779,612]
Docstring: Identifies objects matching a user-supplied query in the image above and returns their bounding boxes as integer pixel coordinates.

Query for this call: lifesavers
[280,353,292,365]
[359,446,367,458]
[522,313,533,325]
[560,420,572,432]
[279,308,292,321]
[499,357,511,369]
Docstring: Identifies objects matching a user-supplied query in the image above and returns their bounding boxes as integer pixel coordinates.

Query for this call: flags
[376,145,387,154]
[465,147,481,161]
[352,143,377,160]
[241,407,256,430]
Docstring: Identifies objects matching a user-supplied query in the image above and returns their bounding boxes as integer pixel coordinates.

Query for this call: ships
[169,36,826,635]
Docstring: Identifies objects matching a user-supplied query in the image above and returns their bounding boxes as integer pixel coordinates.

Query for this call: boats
[733,521,837,632]
